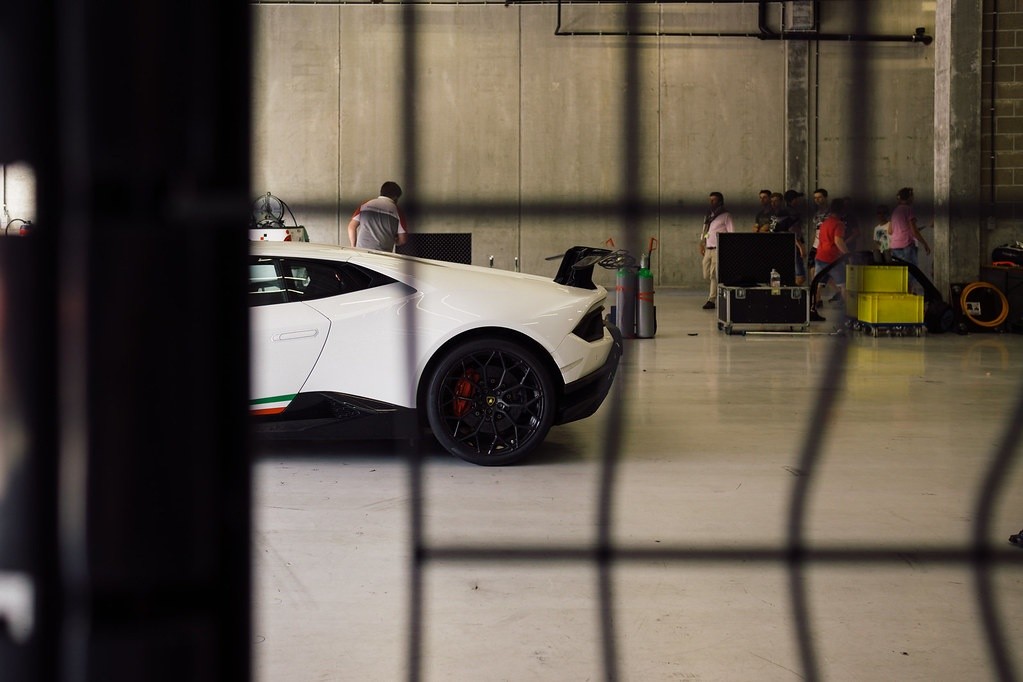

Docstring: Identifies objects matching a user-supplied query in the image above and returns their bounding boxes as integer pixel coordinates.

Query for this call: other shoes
[810,309,826,322]
[816,299,823,308]
[703,301,716,310]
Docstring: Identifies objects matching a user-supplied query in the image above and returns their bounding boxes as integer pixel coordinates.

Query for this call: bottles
[770,268,780,287]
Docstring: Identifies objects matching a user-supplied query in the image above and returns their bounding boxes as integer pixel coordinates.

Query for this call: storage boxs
[715,230,811,333]
[844,265,926,325]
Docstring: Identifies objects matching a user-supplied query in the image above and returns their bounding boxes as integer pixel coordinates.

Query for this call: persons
[700,191,736,309]
[755,188,930,321]
[347,181,408,253]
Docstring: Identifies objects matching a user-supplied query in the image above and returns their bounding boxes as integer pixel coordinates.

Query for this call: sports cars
[247,240,624,469]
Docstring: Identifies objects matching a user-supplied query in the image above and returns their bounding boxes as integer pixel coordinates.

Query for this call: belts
[706,247,717,250]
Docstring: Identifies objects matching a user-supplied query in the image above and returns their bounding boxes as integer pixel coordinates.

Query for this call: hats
[784,189,804,201]
